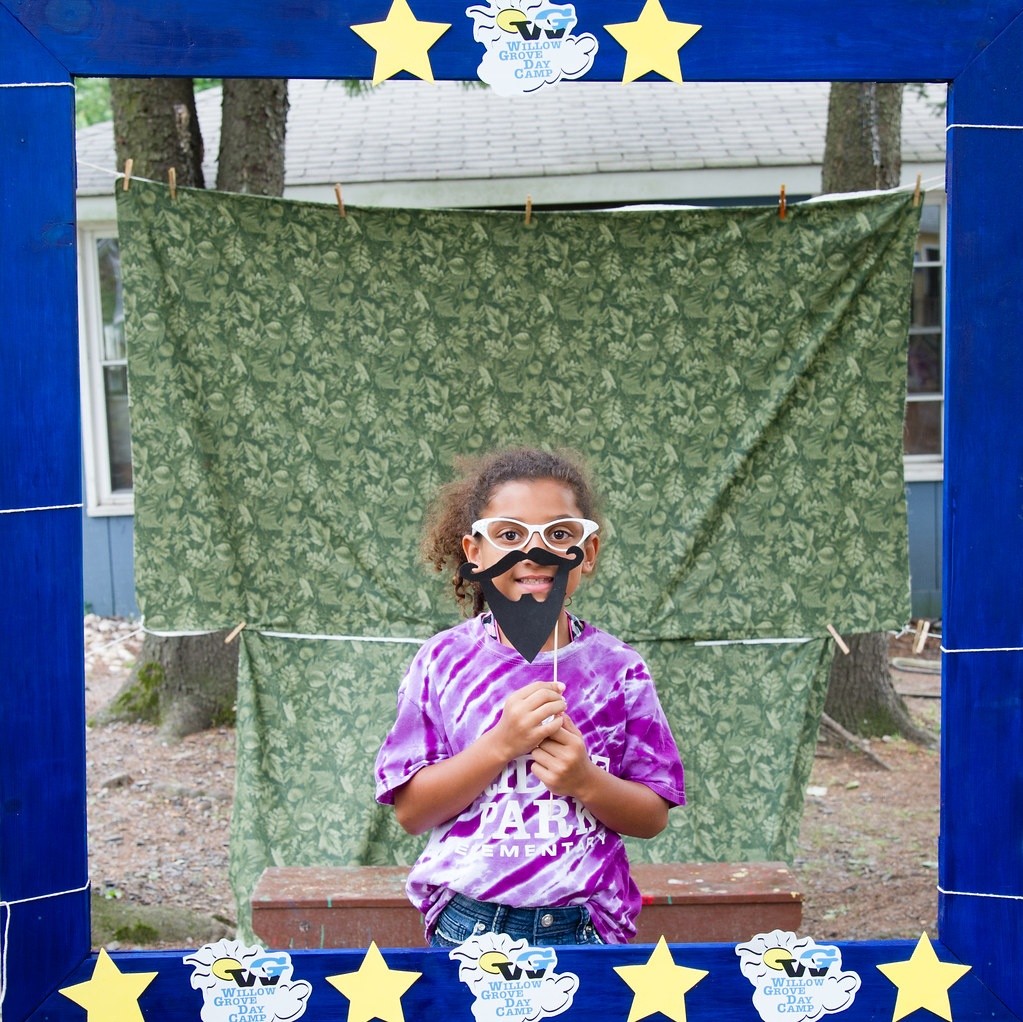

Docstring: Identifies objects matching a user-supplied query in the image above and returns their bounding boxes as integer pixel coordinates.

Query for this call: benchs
[250,860,806,950]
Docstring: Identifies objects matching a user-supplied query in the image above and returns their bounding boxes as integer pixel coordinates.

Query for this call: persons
[373,448,687,948]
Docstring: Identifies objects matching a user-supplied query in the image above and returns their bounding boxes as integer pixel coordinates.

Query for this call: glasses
[471,517,599,552]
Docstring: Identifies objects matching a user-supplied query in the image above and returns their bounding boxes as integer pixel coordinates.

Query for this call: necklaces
[494,619,575,644]
